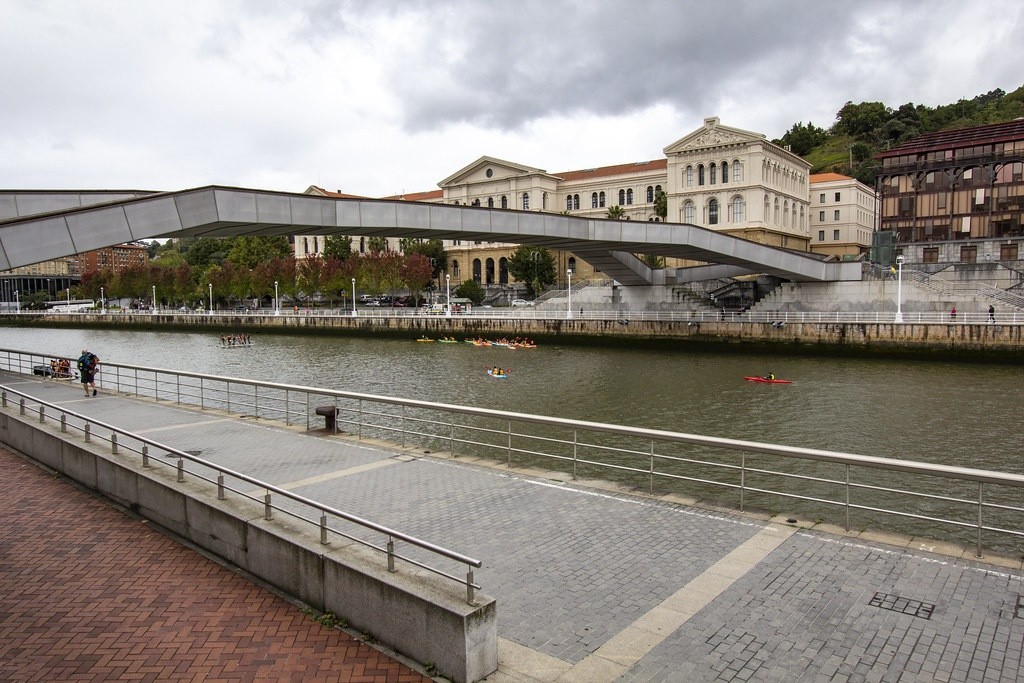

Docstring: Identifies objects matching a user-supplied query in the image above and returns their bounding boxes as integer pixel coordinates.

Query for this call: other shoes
[85,395,89,397]
[93,389,97,396]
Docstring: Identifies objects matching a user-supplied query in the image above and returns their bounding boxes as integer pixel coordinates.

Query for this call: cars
[511,300,535,307]
[360,294,426,307]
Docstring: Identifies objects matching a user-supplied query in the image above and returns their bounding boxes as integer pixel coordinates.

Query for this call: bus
[42,301,94,313]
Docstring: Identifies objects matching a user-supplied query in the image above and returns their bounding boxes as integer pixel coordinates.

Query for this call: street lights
[352,278,356,311]
[152,286,157,314]
[66,289,69,300]
[101,287,106,314]
[895,255,905,322]
[566,269,572,319]
[446,274,450,311]
[209,284,213,314]
[275,281,279,315]
[16,290,19,313]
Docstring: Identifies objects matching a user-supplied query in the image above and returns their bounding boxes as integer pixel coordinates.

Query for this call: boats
[34,366,78,378]
[486,370,506,378]
[438,340,458,343]
[221,343,254,348]
[464,340,537,349]
[417,339,434,341]
[744,376,792,383]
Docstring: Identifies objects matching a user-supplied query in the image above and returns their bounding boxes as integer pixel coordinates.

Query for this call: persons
[15,307,18,313]
[764,372,775,381]
[294,305,299,316]
[50,360,72,379]
[466,336,533,347]
[77,349,97,397]
[491,366,504,376]
[443,336,454,341]
[306,307,309,316]
[950,307,956,322]
[989,305,996,324]
[221,333,251,345]
[581,307,585,319]
[889,266,896,280]
[720,307,726,321]
[423,336,429,340]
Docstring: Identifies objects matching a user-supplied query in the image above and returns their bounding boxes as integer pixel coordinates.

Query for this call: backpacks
[79,352,99,376]
